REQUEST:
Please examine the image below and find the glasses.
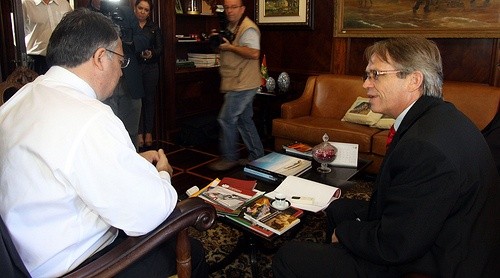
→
[106,48,131,69]
[224,4,243,9]
[361,68,401,81]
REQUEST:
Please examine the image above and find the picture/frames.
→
[333,0,500,38]
[254,0,315,32]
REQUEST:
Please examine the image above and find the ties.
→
[385,123,396,148]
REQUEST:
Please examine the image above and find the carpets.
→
[187,181,374,278]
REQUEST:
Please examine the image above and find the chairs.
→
[0,196,216,278]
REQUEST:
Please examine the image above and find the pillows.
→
[342,96,384,126]
[369,114,396,129]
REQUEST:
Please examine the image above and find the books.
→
[192,138,359,237]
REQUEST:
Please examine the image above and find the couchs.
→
[272,74,500,175]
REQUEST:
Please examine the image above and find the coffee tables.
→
[177,149,373,278]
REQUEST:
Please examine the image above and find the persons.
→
[124,1,162,149]
[82,1,145,155]
[0,8,207,278]
[272,31,500,278]
[208,0,265,171]
[19,0,75,80]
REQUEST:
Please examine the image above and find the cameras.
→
[209,30,234,48]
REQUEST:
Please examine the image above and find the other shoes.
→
[239,158,252,166]
[209,159,237,171]
[136,132,154,148]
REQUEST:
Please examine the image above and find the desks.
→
[255,88,303,144]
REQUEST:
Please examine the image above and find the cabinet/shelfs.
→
[158,0,227,142]
